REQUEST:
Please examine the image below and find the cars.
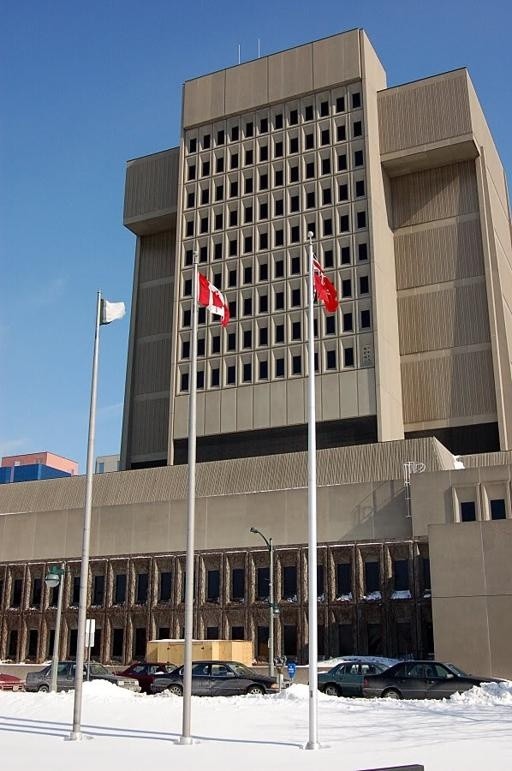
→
[318,661,391,697]
[0,660,290,696]
[362,661,509,699]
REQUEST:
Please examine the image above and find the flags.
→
[198,271,230,327]
[99,299,128,326]
[313,256,340,315]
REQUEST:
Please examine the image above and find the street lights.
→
[250,527,274,676]
[44,559,67,694]
